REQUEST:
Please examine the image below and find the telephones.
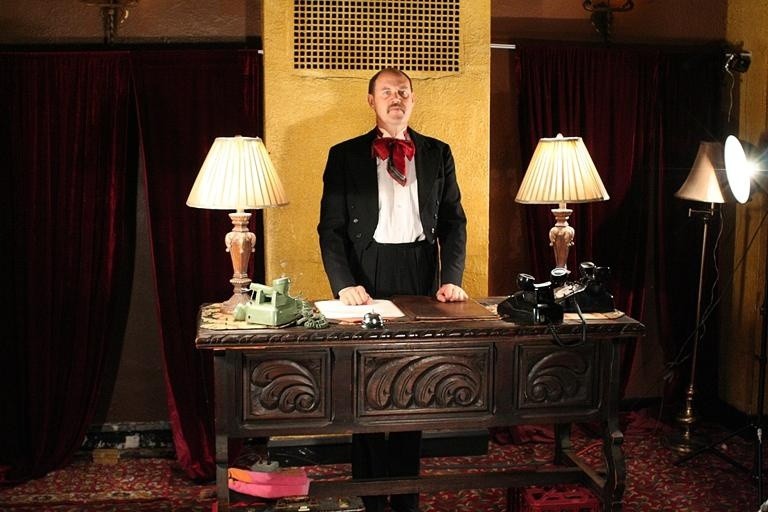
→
[496,262,616,326]
[233,276,302,326]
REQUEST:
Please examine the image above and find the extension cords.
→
[250,458,280,473]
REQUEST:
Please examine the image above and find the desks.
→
[193,291,647,511]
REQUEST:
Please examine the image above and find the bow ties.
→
[373,137,415,186]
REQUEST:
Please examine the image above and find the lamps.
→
[671,135,738,458]
[512,130,614,299]
[186,135,289,314]
[717,136,767,512]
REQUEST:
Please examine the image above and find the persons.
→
[316,67,469,510]
